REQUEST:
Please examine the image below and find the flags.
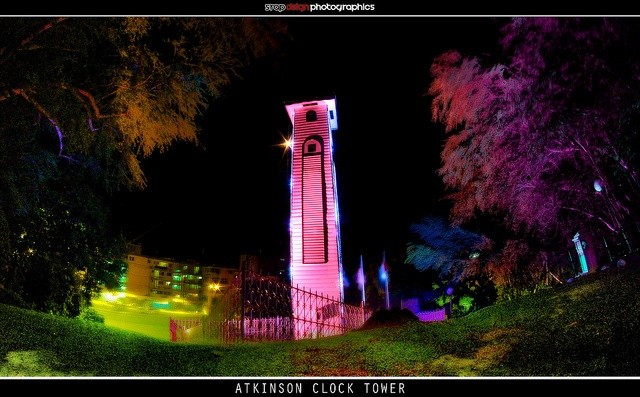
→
[378,257,391,284]
[356,267,367,291]
